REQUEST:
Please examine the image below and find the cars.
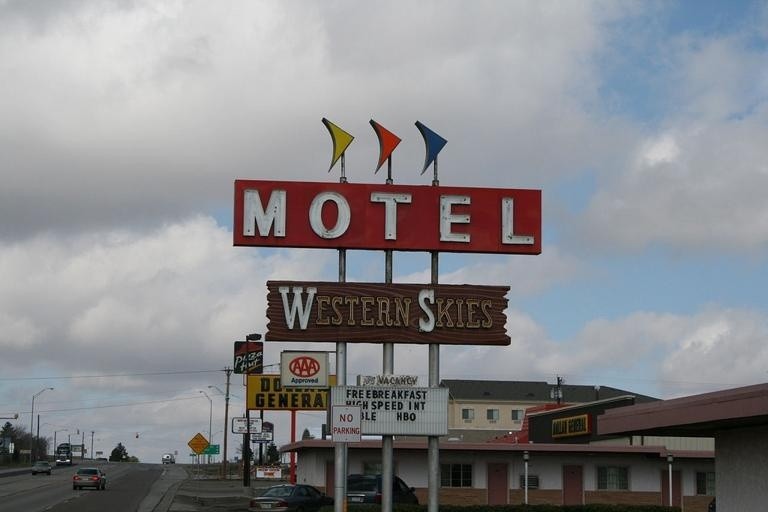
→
[248,484,333,512]
[73,467,107,490]
[31,460,51,475]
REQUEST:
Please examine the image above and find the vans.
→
[161,453,175,465]
[347,473,418,505]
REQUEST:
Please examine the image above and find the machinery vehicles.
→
[53,443,73,466]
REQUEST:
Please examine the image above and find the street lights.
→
[0,412,18,420]
[593,384,600,400]
[666,454,673,507]
[54,428,68,459]
[199,390,213,463]
[28,387,55,465]
[202,429,224,463]
[207,385,226,460]
[521,450,530,504]
